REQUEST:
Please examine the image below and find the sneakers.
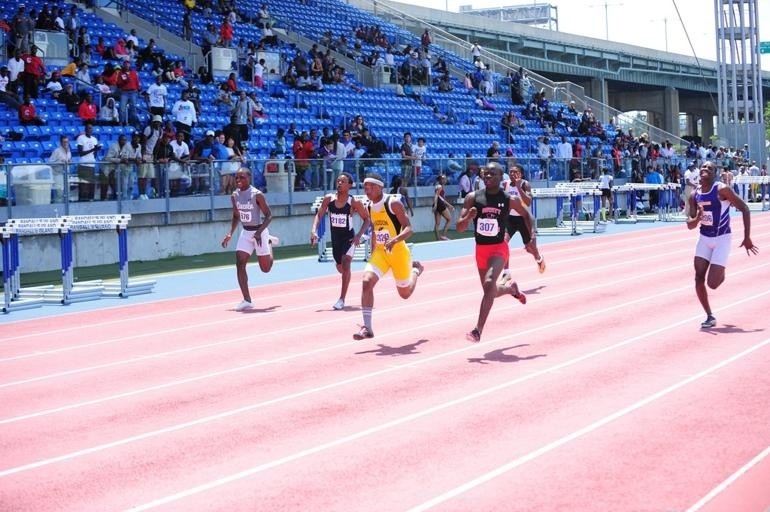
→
[333,298,345,310]
[497,272,512,285]
[701,315,717,328]
[234,300,255,311]
[269,235,280,246]
[137,194,149,200]
[509,280,527,305]
[465,328,483,342]
[353,326,374,340]
[440,235,451,242]
[413,260,425,277]
[536,255,546,273]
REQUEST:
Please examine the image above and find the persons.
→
[431,174,456,240]
[686,161,759,329]
[455,162,537,343]
[2,1,770,220]
[310,173,370,309]
[222,167,278,312]
[353,171,424,340]
[501,165,546,274]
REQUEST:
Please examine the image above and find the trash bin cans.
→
[263,161,298,192]
[12,166,55,206]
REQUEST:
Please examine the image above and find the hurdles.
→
[0,214,156,313]
[529,182,610,235]
[731,175,770,211]
[311,194,405,262]
[611,182,688,224]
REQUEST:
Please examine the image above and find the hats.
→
[206,130,215,137]
[151,114,163,124]
[132,131,142,136]
